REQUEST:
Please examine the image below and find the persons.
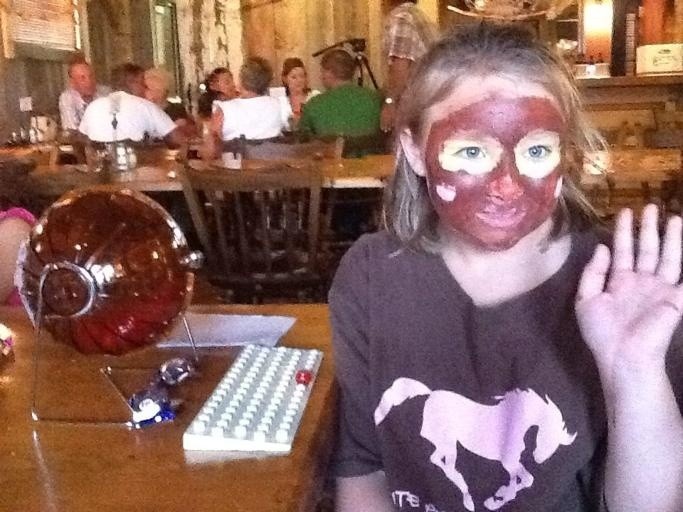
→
[59,60,112,131]
[198,67,235,120]
[379,0,438,134]
[211,56,285,141]
[143,65,189,126]
[281,57,323,117]
[0,158,38,307]
[77,63,182,150]
[327,19,683,512]
[296,50,383,159]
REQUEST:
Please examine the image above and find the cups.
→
[222,150,243,171]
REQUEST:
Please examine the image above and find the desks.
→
[1,302,340,512]
[28,148,683,196]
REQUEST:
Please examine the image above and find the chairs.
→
[239,133,344,248]
[174,152,323,303]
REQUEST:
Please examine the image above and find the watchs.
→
[383,96,395,105]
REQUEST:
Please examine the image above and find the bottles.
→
[575,53,605,64]
